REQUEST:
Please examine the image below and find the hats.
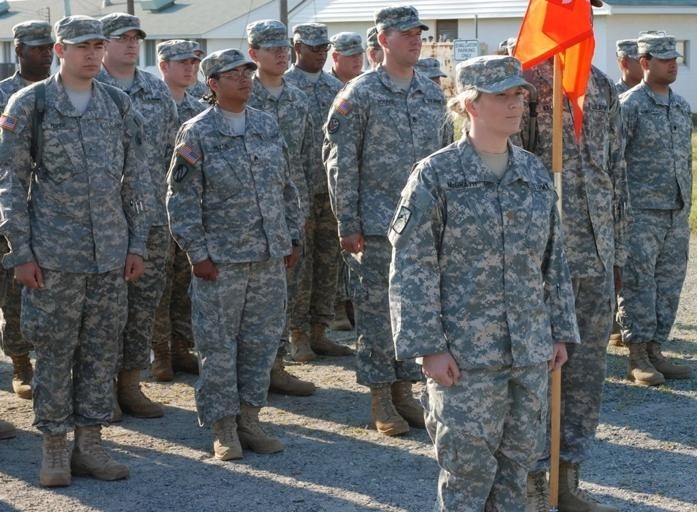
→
[637,31,683,59]
[54,14,111,46]
[616,39,639,60]
[412,58,448,78]
[367,25,382,48]
[157,39,201,61]
[12,21,56,48]
[375,5,429,31]
[188,40,204,56]
[201,48,257,79]
[292,23,334,46]
[247,19,294,48]
[330,32,365,56]
[99,12,147,41]
[455,55,536,94]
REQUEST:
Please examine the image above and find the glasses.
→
[108,34,144,46]
[298,42,331,53]
[216,68,255,79]
[253,45,291,53]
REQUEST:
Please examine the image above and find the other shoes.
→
[0,419,16,439]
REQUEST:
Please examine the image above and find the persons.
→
[388,1,696,511]
[0,5,454,487]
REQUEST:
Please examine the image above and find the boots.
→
[38,434,72,486]
[527,469,549,508]
[646,341,694,379]
[608,318,623,345]
[11,355,35,398]
[212,415,244,460]
[547,460,601,508]
[288,332,314,363]
[150,341,174,382]
[235,403,285,455]
[627,342,666,385]
[310,322,351,357]
[71,424,129,481]
[369,384,410,435]
[117,369,165,418]
[269,348,316,397]
[171,337,200,375]
[389,381,426,429]
[105,381,123,422]
[331,304,353,332]
